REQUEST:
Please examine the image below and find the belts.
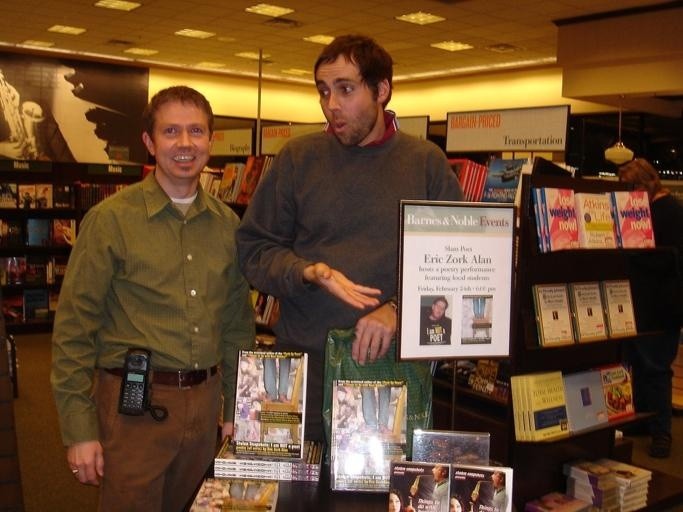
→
[111,367,219,388]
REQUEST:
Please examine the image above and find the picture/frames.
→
[394,198,518,358]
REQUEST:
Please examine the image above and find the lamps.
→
[603,96,635,167]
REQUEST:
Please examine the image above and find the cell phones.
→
[119,349,153,417]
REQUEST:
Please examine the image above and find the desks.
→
[180,436,683,512]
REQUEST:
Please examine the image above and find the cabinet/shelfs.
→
[515,168,659,444]
[186,151,561,344]
[1,157,145,334]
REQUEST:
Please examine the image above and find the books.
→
[532,280,638,347]
[191,351,513,512]
[0,182,128,323]
[510,363,635,442]
[448,157,655,252]
[523,459,653,511]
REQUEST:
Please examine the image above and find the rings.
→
[72,469,78,474]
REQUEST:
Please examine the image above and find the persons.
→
[421,297,451,345]
[50,87,254,512]
[618,158,683,458]
[237,35,465,461]
